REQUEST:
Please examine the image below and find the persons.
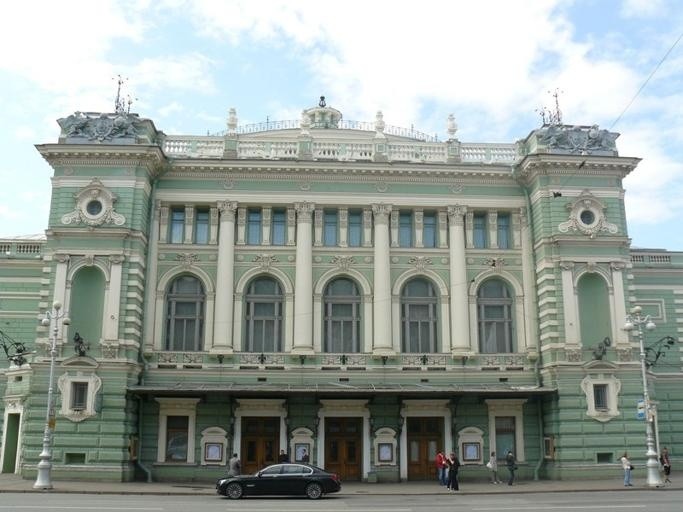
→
[506,451,516,486]
[296,448,306,461]
[489,451,503,485]
[435,448,461,492]
[620,451,634,487]
[659,446,672,484]
[228,453,241,477]
[279,449,289,464]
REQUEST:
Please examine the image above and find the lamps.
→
[491,260,496,268]
[553,192,561,198]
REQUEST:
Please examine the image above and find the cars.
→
[166,432,187,461]
[215,462,341,500]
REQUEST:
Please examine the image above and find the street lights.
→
[622,304,666,488]
[33,300,71,490]
